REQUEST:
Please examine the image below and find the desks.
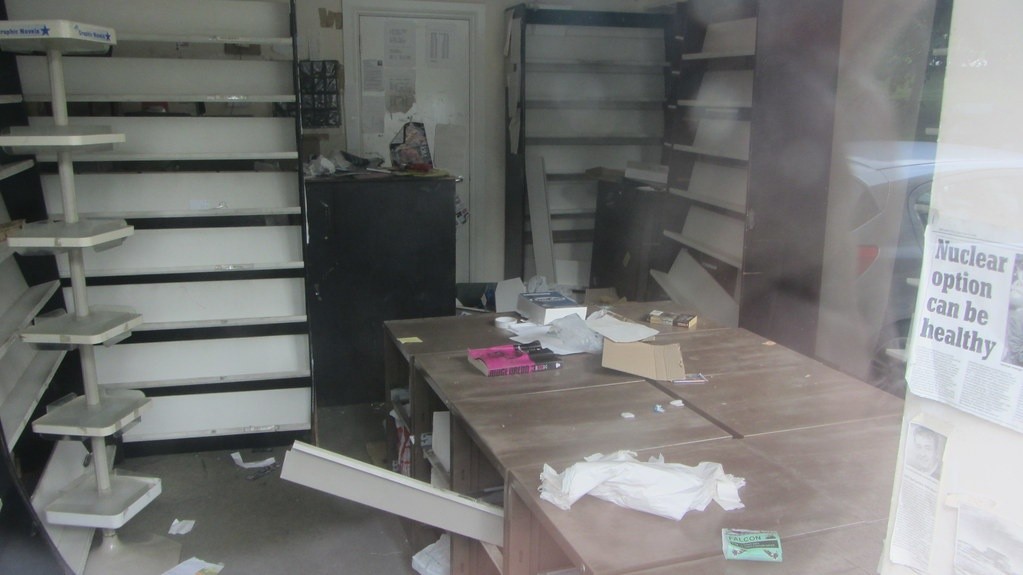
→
[383,300,1023,575]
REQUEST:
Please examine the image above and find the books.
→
[466,340,562,378]
[513,290,588,325]
[645,308,697,327]
[624,159,670,184]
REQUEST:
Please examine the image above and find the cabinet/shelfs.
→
[18,38,311,443]
[503,4,684,295]
[305,170,456,406]
[651,15,759,326]
[0,94,120,575]
[589,178,689,302]
[0,17,184,575]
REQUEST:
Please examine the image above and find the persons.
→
[905,427,943,480]
